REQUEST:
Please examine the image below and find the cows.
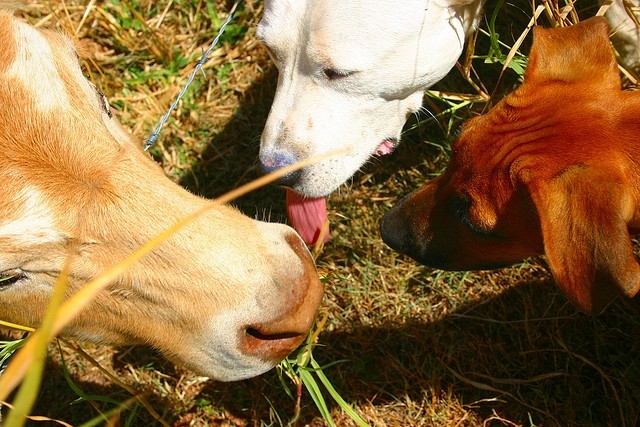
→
[0,10,324,384]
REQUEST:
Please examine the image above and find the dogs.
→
[256,0,482,246]
[380,16,640,317]
[606,0,640,74]
